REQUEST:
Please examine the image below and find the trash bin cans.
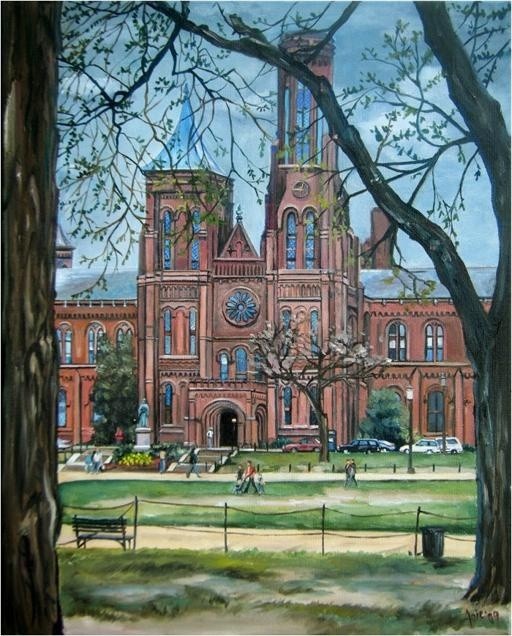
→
[420,526,444,558]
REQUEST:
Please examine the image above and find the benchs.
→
[72,514,135,550]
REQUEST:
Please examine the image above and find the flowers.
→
[119,453,154,468]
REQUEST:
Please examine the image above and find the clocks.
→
[291,179,310,199]
